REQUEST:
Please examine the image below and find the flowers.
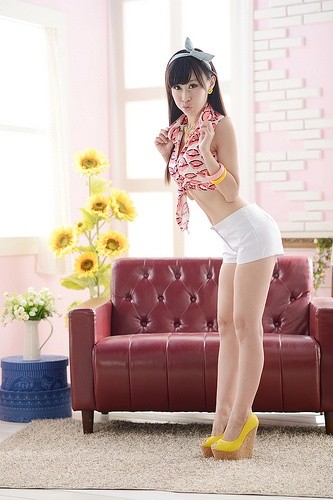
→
[40,148,138,333]
[0,286,65,328]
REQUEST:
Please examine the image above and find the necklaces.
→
[185,123,195,133]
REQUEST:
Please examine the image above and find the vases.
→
[23,318,53,362]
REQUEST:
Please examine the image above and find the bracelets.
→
[206,163,227,184]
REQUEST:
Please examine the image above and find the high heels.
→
[200,430,225,458]
[211,414,259,460]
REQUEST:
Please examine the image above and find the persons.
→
[155,37,284,459]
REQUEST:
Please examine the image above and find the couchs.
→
[69,258,333,435]
